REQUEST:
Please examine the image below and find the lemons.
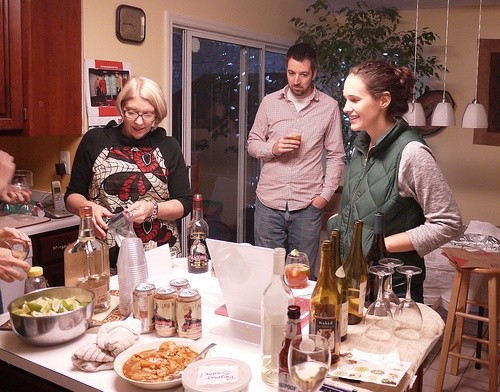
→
[12,297,87,317]
[290,248,300,257]
[300,267,309,273]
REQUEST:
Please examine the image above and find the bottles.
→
[25,266,46,295]
[261,246,296,385]
[278,305,308,392]
[343,219,368,326]
[63,206,110,314]
[309,228,347,365]
[187,193,209,274]
[365,211,391,308]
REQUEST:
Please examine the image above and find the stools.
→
[435,258,500,392]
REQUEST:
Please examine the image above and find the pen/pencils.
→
[324,381,358,392]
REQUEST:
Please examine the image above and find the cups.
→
[285,252,309,289]
[0,199,36,221]
[181,356,253,392]
[0,237,30,261]
[8,169,34,192]
[116,238,150,316]
[285,129,301,150]
[287,335,331,392]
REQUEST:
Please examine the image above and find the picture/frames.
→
[472,38,500,148]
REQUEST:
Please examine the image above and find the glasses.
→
[123,108,157,123]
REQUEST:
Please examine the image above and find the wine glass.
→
[363,258,423,342]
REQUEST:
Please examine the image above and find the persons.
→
[326,60,463,304]
[0,149,32,283]
[94,73,127,106]
[247,42,345,281]
[64,77,195,277]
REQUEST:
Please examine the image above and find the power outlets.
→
[60,149,71,173]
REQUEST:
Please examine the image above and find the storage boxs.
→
[208,291,313,347]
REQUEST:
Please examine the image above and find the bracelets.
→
[146,198,158,222]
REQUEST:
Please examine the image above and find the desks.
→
[423,220,500,369]
[0,232,446,392]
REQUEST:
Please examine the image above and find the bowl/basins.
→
[6,286,95,346]
[113,338,210,390]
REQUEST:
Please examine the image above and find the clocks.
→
[115,3,147,43]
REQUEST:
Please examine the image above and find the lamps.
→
[430,0,457,126]
[401,0,426,127]
[462,0,488,129]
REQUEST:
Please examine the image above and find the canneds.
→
[133,278,203,340]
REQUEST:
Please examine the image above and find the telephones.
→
[44,181,75,219]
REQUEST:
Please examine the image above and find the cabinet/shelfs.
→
[30,226,79,287]
[0,0,83,134]
